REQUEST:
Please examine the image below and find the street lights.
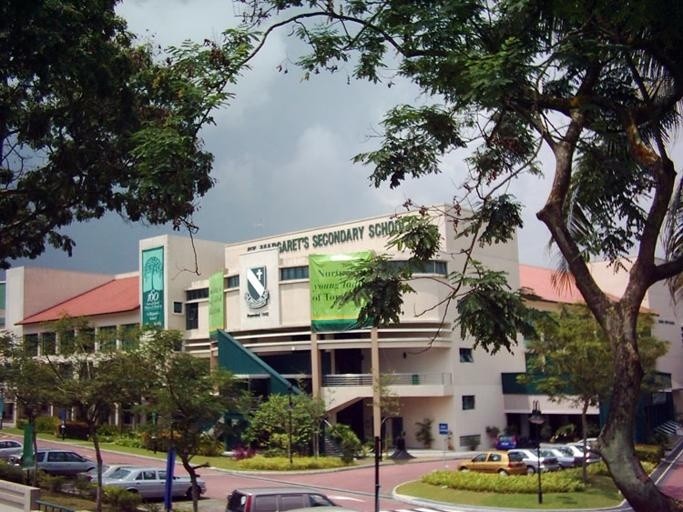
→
[373,413,416,511]
[529,399,544,503]
[284,382,293,464]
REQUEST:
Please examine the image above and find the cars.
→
[458,431,602,476]
[0,438,206,501]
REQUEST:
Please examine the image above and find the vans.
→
[225,487,336,511]
